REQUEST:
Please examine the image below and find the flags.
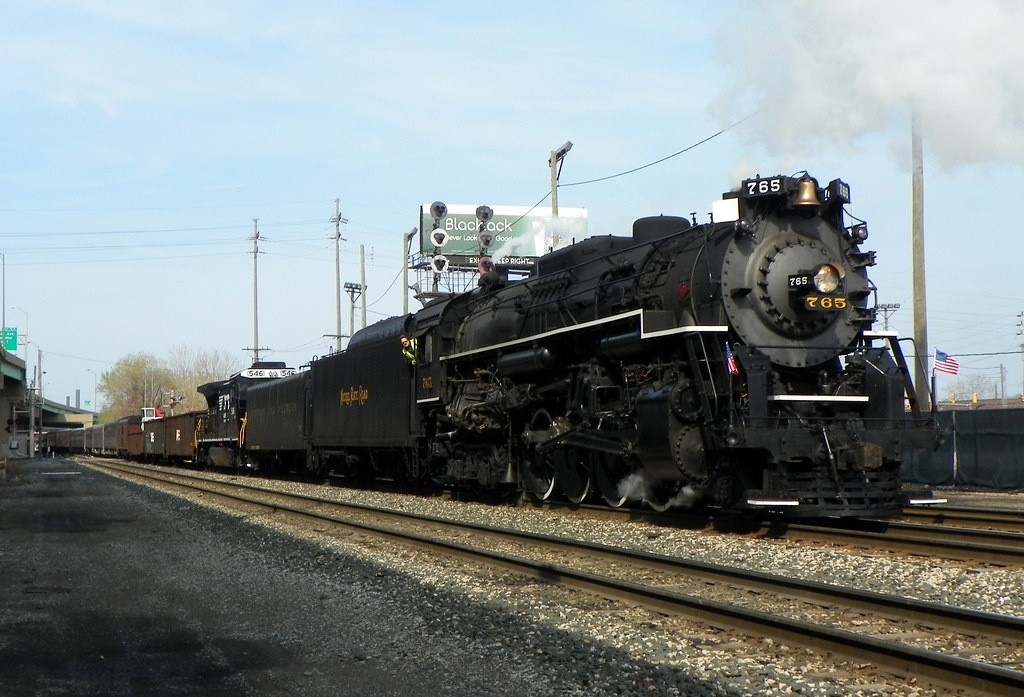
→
[934,348,959,375]
[726,342,739,375]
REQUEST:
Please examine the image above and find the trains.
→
[43,170,956,539]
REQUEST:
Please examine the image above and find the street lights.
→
[87,366,96,413]
[11,306,29,360]
